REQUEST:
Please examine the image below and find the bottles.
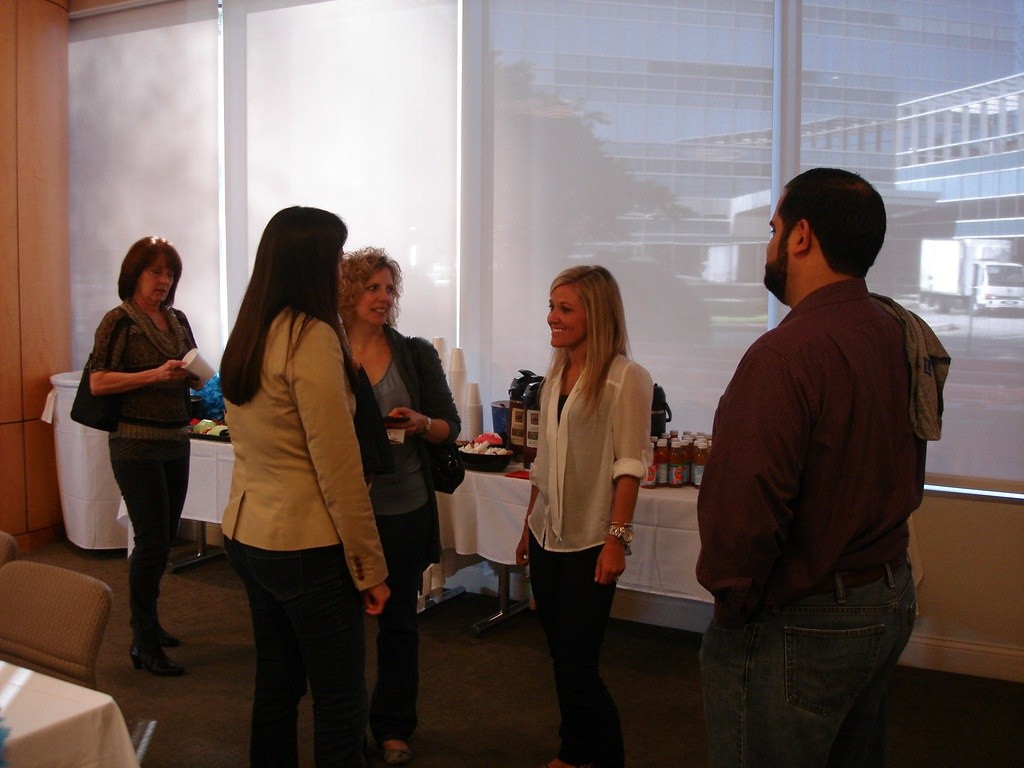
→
[632,429,715,490]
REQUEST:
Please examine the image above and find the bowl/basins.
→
[456,444,516,473]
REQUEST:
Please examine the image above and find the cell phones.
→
[381,415,411,423]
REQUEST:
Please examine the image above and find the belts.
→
[809,552,907,594]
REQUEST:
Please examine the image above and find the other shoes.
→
[380,739,413,765]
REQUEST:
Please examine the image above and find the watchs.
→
[609,526,634,543]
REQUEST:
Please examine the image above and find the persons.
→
[220,208,390,768]
[696,168,927,768]
[89,237,206,674]
[517,266,654,768]
[338,251,462,764]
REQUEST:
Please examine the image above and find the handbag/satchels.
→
[71,307,129,433]
[429,442,465,495]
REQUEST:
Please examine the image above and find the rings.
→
[615,576,620,582]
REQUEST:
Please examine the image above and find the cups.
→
[181,348,215,381]
[431,336,484,444]
[490,400,511,444]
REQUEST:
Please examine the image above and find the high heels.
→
[130,617,179,647]
[130,637,183,677]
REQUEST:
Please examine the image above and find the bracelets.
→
[611,521,633,528]
[415,417,432,436]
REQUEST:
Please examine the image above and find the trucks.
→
[917,237,1024,318]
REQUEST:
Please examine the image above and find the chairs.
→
[0,531,112,687]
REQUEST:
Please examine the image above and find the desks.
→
[0,662,141,767]
[115,435,1023,686]
